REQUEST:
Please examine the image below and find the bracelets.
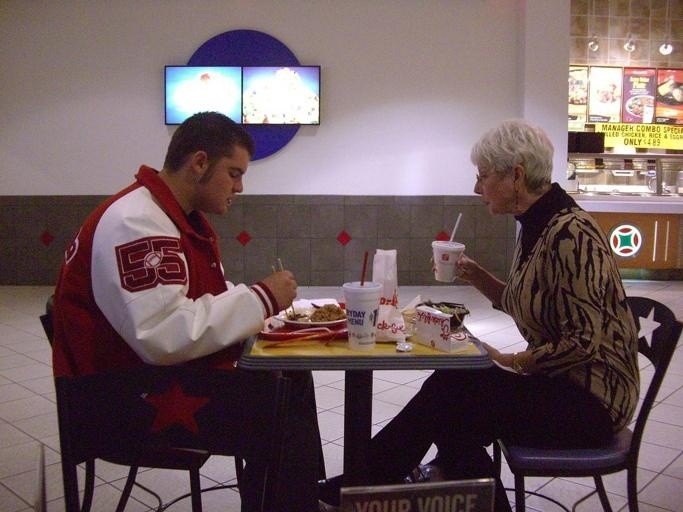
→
[512,352,525,374]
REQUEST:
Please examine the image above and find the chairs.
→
[40,295,245,511]
[493,296,683,511]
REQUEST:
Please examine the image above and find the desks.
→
[248,309,495,471]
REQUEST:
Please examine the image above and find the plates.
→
[624,95,654,119]
[272,304,352,326]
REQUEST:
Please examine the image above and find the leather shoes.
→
[318,474,343,506]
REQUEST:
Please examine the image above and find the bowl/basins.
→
[415,303,470,328]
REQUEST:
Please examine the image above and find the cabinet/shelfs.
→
[568,151,682,272]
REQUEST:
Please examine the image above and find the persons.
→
[53,112,326,512]
[313,118,640,512]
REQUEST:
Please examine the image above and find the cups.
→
[342,280,383,351]
[431,240,465,282]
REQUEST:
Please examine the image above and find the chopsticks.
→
[270,257,296,321]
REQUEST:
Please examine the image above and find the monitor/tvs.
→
[241,64,322,126]
[164,64,242,127]
[568,131,604,166]
